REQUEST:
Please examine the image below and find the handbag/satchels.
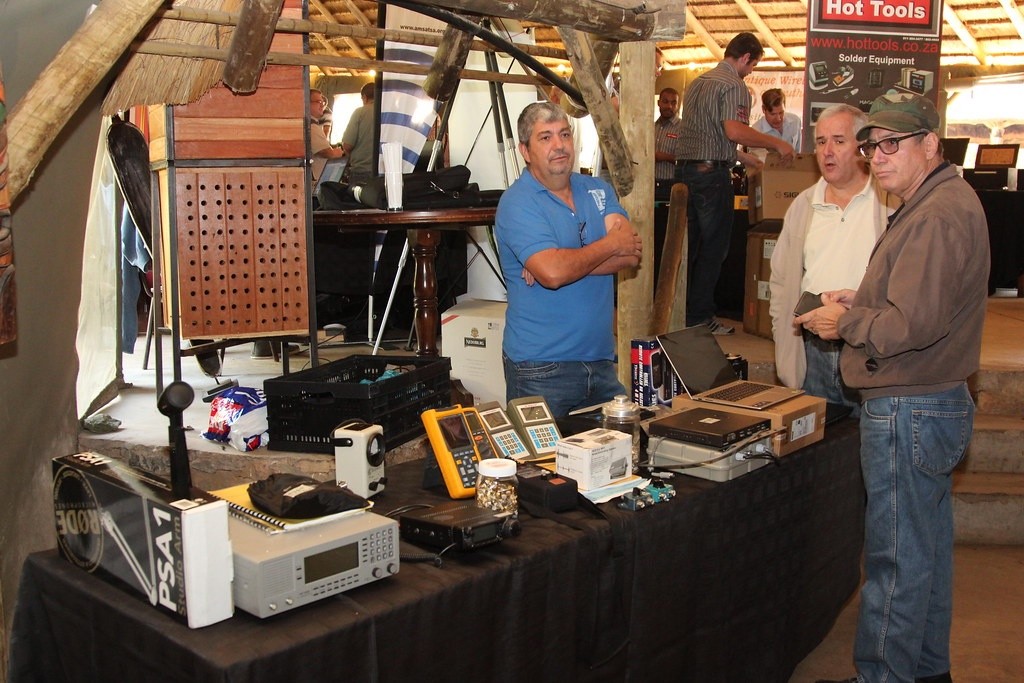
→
[201,386,269,453]
[320,164,483,210]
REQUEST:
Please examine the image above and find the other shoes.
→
[814,678,858,683]
[914,671,953,683]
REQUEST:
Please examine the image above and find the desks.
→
[313,207,498,374]
[973,190,1024,297]
[11,403,866,683]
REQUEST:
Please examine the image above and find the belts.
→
[675,160,731,170]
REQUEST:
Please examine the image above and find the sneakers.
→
[686,315,735,335]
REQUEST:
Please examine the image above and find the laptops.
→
[656,323,806,410]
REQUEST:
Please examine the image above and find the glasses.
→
[857,131,929,160]
[310,99,326,108]
[577,221,588,249]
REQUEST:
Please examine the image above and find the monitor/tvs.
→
[940,136,970,166]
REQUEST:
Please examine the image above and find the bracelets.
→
[675,161,677,165]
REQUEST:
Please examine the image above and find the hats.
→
[856,93,940,142]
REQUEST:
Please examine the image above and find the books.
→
[206,482,374,535]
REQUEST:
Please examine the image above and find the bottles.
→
[475,457,520,539]
[601,394,641,473]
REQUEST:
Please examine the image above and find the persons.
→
[769,105,901,420]
[740,89,802,176]
[342,82,375,185]
[794,92,990,683]
[654,88,682,298]
[674,32,798,335]
[309,88,342,180]
[494,103,643,410]
[612,47,663,112]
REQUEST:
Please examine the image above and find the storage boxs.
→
[672,391,827,458]
[264,355,452,456]
[744,153,903,340]
[557,429,632,491]
[441,294,507,410]
[52,451,236,630]
